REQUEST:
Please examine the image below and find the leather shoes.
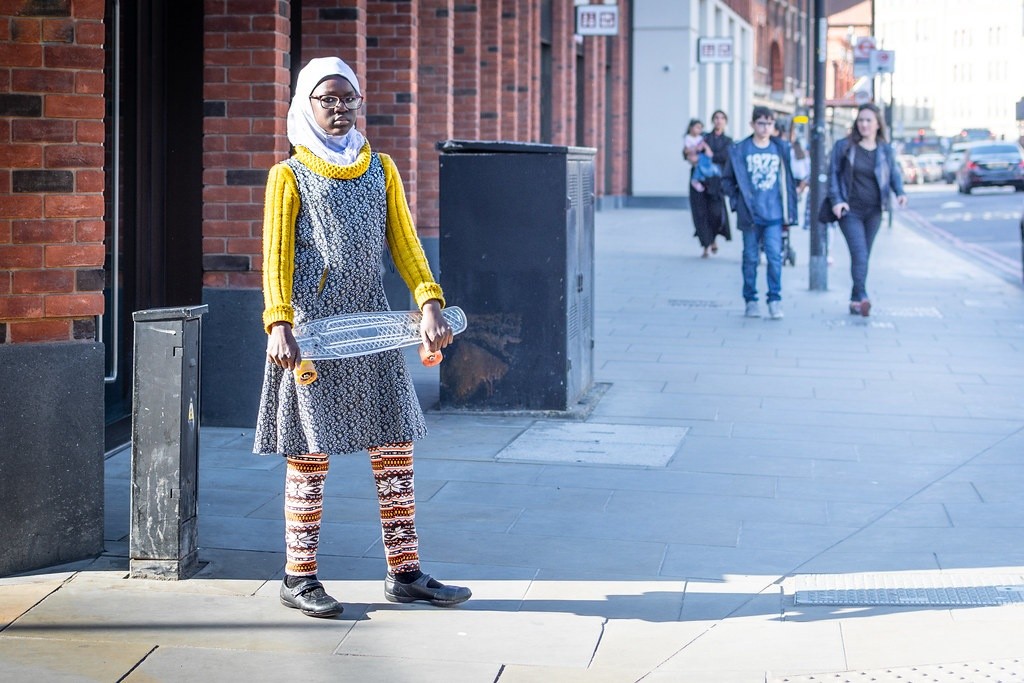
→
[383,568,472,608]
[280,573,343,617]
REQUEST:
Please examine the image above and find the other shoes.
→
[849,302,862,315]
[745,301,761,318]
[698,251,709,258]
[860,298,871,316]
[768,301,783,319]
[710,241,719,253]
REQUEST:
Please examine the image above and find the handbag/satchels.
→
[819,197,840,223]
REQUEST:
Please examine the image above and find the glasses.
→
[756,121,774,127]
[306,95,363,112]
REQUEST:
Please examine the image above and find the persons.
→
[681,117,714,192]
[760,118,812,267]
[825,103,908,317]
[251,56,471,618]
[682,111,735,259]
[718,106,800,321]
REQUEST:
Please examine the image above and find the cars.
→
[895,153,947,183]
[958,141,1024,194]
[945,143,970,183]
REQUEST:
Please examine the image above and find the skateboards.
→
[781,226,796,267]
[291,305,468,385]
